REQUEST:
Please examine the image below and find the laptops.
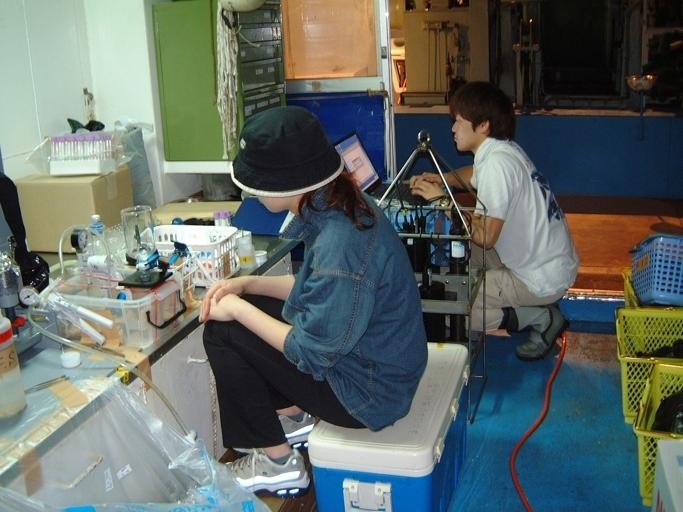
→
[331,131,428,202]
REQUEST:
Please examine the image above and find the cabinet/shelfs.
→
[151,0,285,159]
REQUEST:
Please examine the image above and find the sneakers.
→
[232,411,314,454]
[516,304,565,361]
[221,451,310,497]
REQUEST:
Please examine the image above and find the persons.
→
[409,82,578,360]
[199,106,427,499]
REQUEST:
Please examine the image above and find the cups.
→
[118,204,158,265]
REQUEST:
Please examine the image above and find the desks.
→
[0,234,303,512]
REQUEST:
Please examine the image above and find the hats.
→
[231,106,343,197]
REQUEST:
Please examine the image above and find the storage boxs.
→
[649,439,682,512]
[305,343,471,512]
[48,266,186,349]
[15,162,134,252]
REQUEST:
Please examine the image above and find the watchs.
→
[439,198,450,213]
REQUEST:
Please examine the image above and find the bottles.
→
[88,214,109,257]
[213,210,232,228]
[50,134,113,161]
[238,237,255,268]
[0,309,26,419]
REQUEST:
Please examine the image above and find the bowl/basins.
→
[626,75,656,91]
